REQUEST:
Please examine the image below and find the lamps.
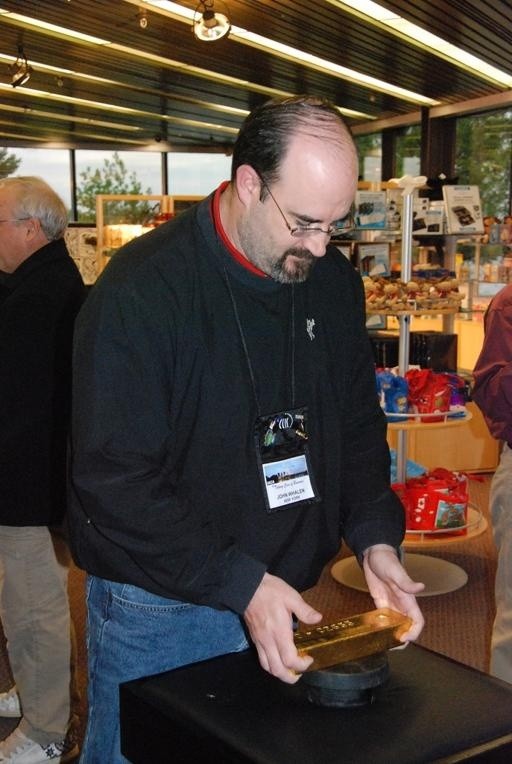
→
[190,0,232,45]
[7,42,34,87]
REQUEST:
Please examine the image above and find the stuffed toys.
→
[355,273,466,320]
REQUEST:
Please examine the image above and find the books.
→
[337,176,486,278]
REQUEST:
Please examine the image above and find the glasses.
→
[254,166,357,241]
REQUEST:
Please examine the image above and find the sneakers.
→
[1,727,80,764]
[0,683,23,718]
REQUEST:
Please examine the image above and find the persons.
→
[1,173,91,764]
[59,92,426,764]
[468,276,510,684]
